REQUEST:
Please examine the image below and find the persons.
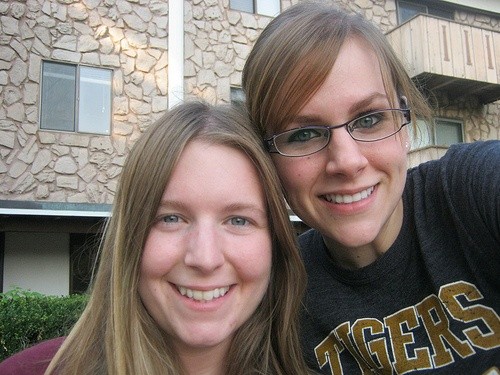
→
[241,0,500,375]
[0,101,320,375]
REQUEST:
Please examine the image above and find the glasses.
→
[263,106,411,158]
